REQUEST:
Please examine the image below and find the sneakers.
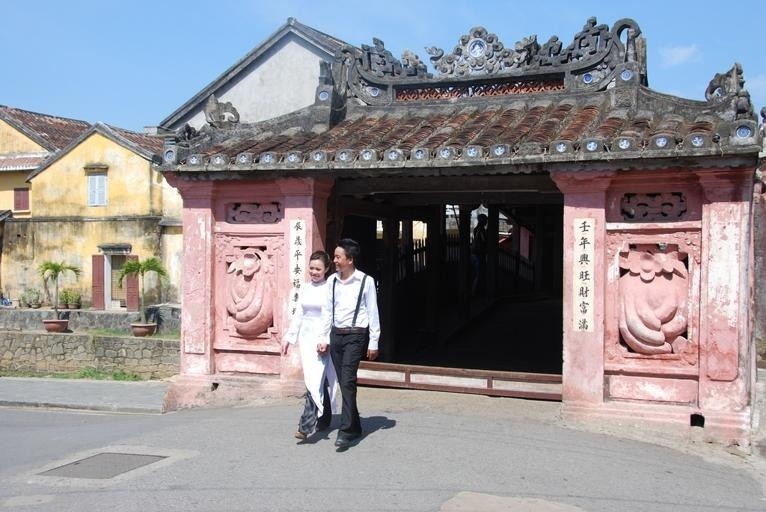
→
[295,431,307,440]
[314,420,328,431]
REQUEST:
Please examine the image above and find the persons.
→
[470,214,495,298]
[317,239,381,447]
[279,251,334,441]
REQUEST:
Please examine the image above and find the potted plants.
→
[116,255,168,336]
[12,258,83,332]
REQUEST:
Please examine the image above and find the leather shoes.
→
[335,437,352,447]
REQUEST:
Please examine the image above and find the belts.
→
[333,327,368,335]
